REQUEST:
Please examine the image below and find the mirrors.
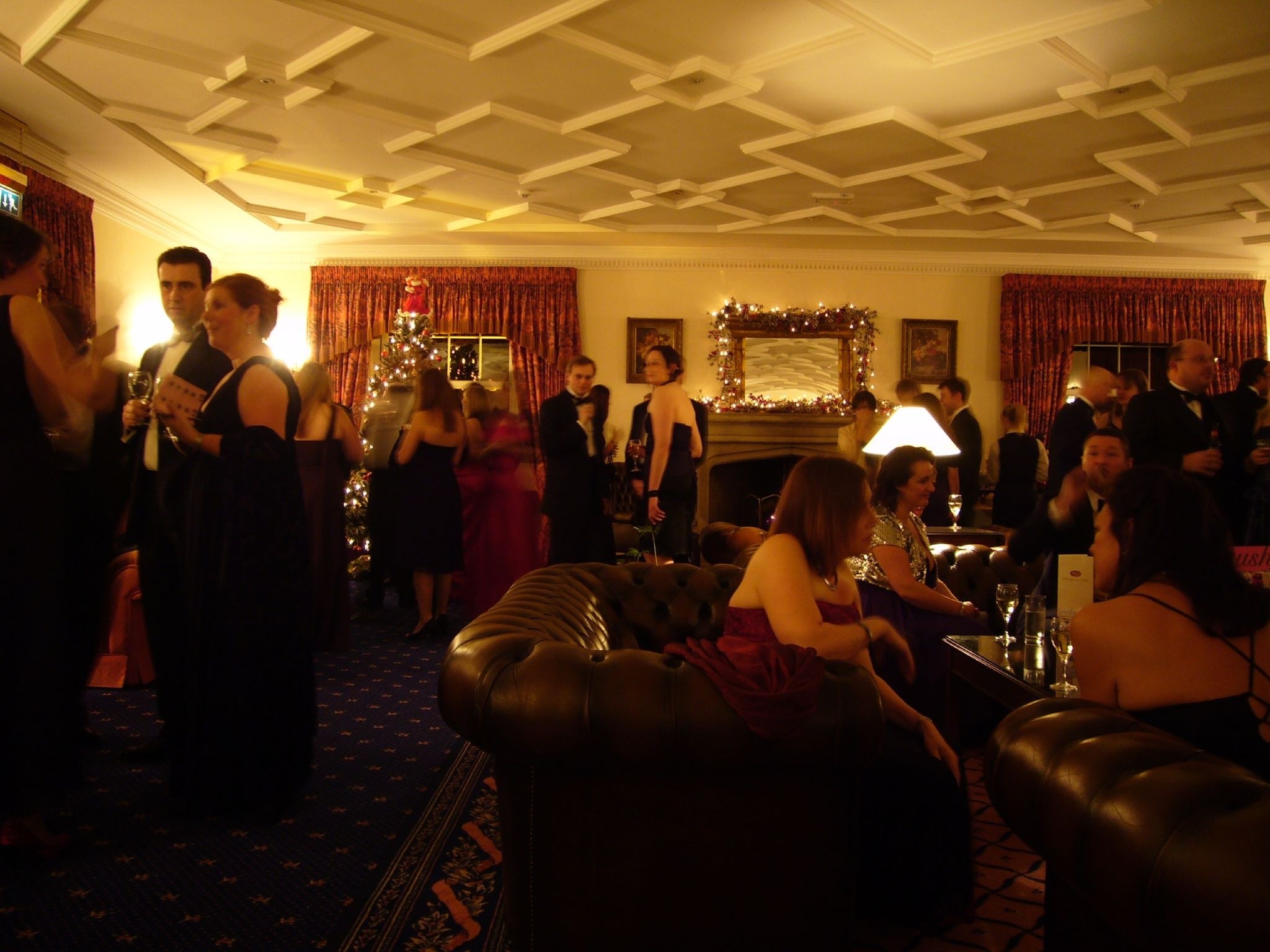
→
[742,337,839,403]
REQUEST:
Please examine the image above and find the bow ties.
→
[573,396,591,405]
[1178,390,1208,403]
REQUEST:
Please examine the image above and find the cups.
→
[1024,595,1046,647]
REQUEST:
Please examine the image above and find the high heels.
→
[405,617,436,643]
[436,615,448,629]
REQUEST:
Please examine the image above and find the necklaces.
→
[825,570,837,589]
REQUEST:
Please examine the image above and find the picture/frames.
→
[902,318,958,384]
[627,317,683,383]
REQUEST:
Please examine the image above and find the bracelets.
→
[194,431,204,452]
[645,489,661,498]
[856,620,875,644]
[914,716,933,729]
[959,601,966,616]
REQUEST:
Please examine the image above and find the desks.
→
[924,525,1007,547]
[941,634,1053,739]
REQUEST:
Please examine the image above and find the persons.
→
[155,273,311,823]
[663,457,972,930]
[835,339,1270,636]
[624,341,708,567]
[290,362,366,655]
[356,368,543,640]
[1071,460,1270,778]
[530,354,621,568]
[121,247,234,826]
[845,446,990,726]
[0,202,129,884]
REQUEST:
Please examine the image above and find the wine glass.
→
[127,371,153,427]
[995,584,1019,643]
[1257,441,1266,465]
[1050,616,1078,692]
[948,494,962,530]
[629,439,641,472]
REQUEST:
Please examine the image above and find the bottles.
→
[1207,421,1221,476]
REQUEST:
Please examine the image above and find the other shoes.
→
[118,741,175,764]
[358,590,384,606]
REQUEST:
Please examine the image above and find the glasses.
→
[1174,355,1215,365]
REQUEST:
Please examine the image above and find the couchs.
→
[986,699,1270,952]
[438,562,886,952]
[928,544,1008,613]
